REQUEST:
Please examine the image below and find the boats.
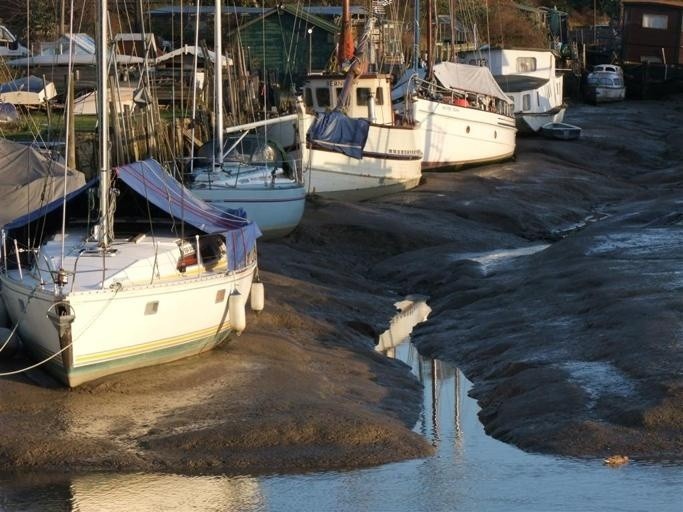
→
[586,62,626,103]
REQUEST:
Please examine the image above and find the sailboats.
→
[187,0,305,241]
[1,46,265,389]
[260,1,423,190]
[455,48,565,135]
[383,0,517,171]
